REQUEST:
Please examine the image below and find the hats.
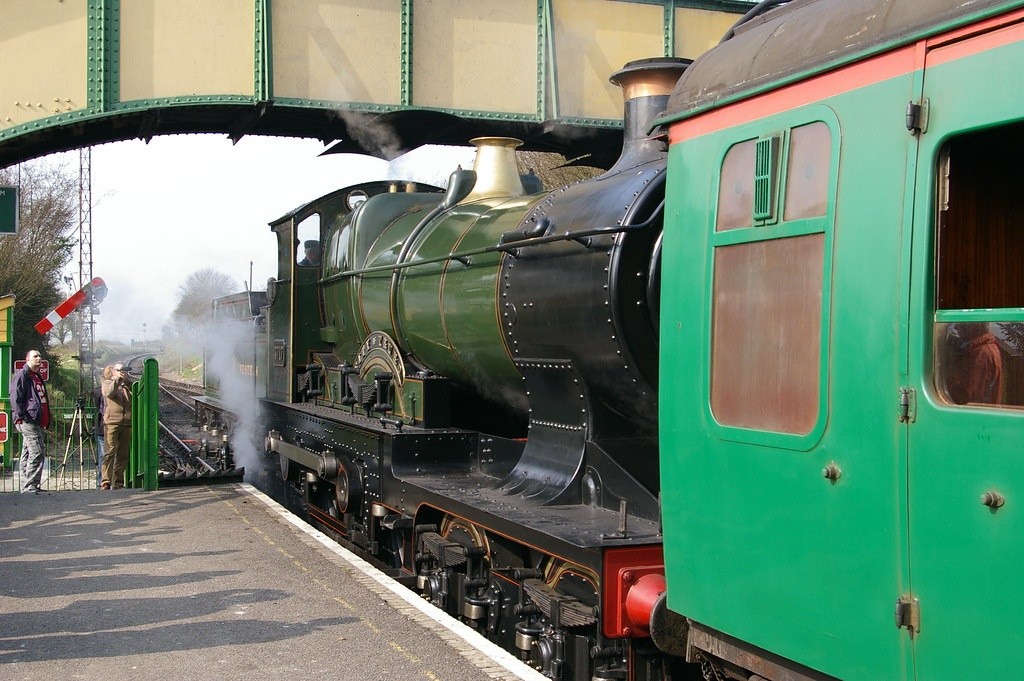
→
[304,240,318,248]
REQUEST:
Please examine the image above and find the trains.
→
[197,1,1022,680]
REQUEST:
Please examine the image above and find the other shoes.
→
[101,484,125,490]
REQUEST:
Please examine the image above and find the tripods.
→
[57,359,99,493]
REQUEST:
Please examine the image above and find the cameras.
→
[119,366,132,382]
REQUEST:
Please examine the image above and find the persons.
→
[937,321,1002,406]
[93,362,133,490]
[10,350,51,493]
